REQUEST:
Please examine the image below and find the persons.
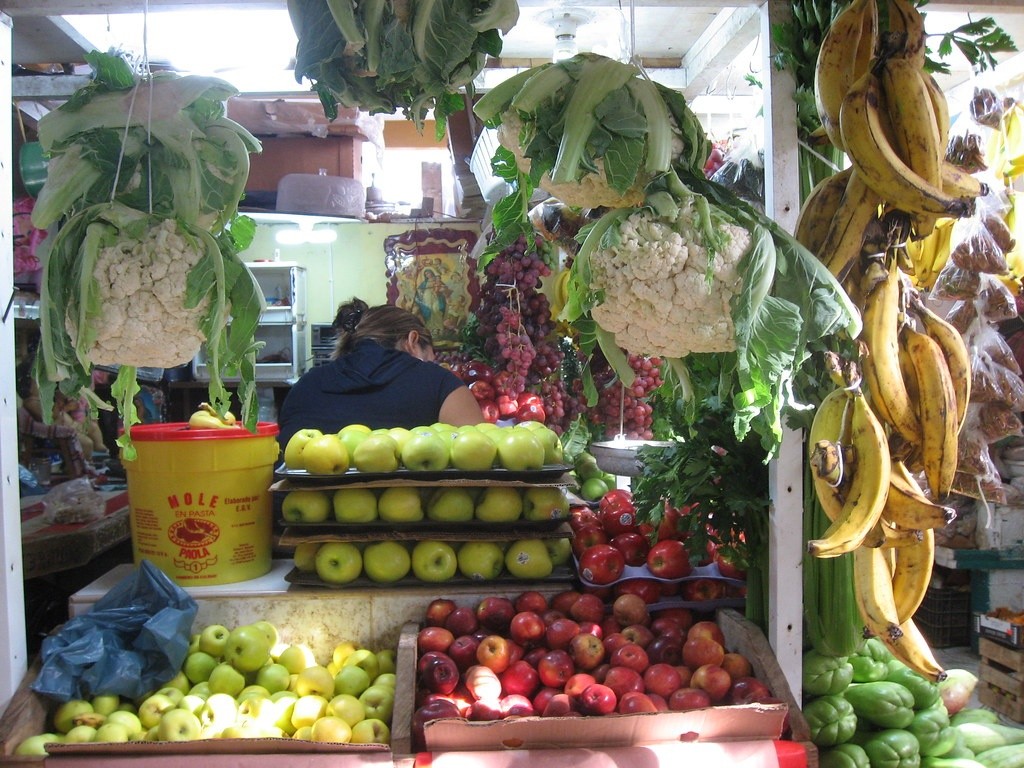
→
[274,296,484,455]
[16,375,108,465]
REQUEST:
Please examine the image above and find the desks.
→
[937,545,1024,657]
[19,484,132,580]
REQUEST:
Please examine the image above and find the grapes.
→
[434,232,663,441]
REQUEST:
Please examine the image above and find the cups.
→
[29,456,53,486]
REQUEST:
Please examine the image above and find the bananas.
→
[991,103,1024,298]
[796,0,988,683]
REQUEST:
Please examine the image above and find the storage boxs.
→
[913,585,973,649]
[241,135,363,188]
[977,613,1024,722]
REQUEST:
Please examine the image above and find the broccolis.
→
[496,94,685,212]
[587,208,754,357]
[48,129,227,370]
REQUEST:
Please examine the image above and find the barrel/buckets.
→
[116,415,279,590]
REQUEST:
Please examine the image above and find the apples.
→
[13,364,790,755]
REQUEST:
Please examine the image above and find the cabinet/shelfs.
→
[193,262,307,381]
